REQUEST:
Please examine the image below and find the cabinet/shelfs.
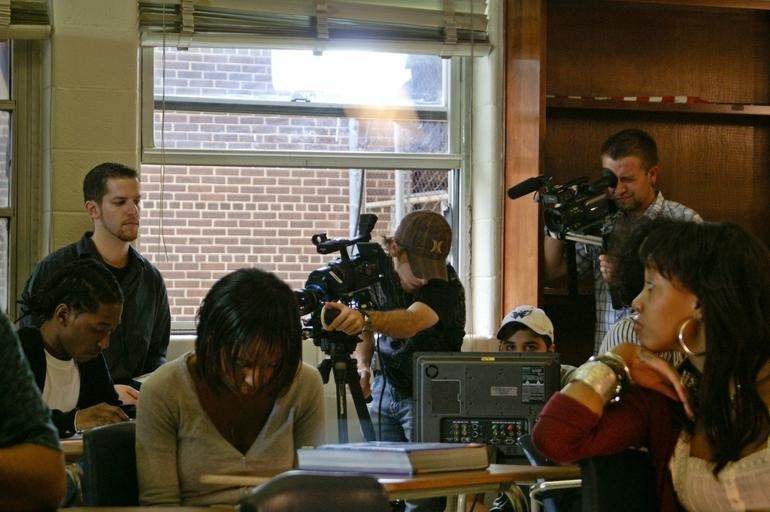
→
[502,2,770,326]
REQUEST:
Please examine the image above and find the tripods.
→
[317,356,375,444]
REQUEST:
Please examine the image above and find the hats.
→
[395,210,452,282]
[497,305,555,345]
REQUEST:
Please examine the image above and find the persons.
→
[322,210,467,509]
[17,161,171,410]
[532,220,769,511]
[542,128,706,369]
[136,270,327,512]
[0,306,68,511]
[459,306,581,511]
[2,254,136,442]
[594,212,698,389]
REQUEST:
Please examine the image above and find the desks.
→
[195,459,580,512]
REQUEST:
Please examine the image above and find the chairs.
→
[241,470,388,510]
[580,452,655,510]
[84,421,140,505]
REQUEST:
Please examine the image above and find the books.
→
[292,439,490,478]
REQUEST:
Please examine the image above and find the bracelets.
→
[566,348,635,406]
